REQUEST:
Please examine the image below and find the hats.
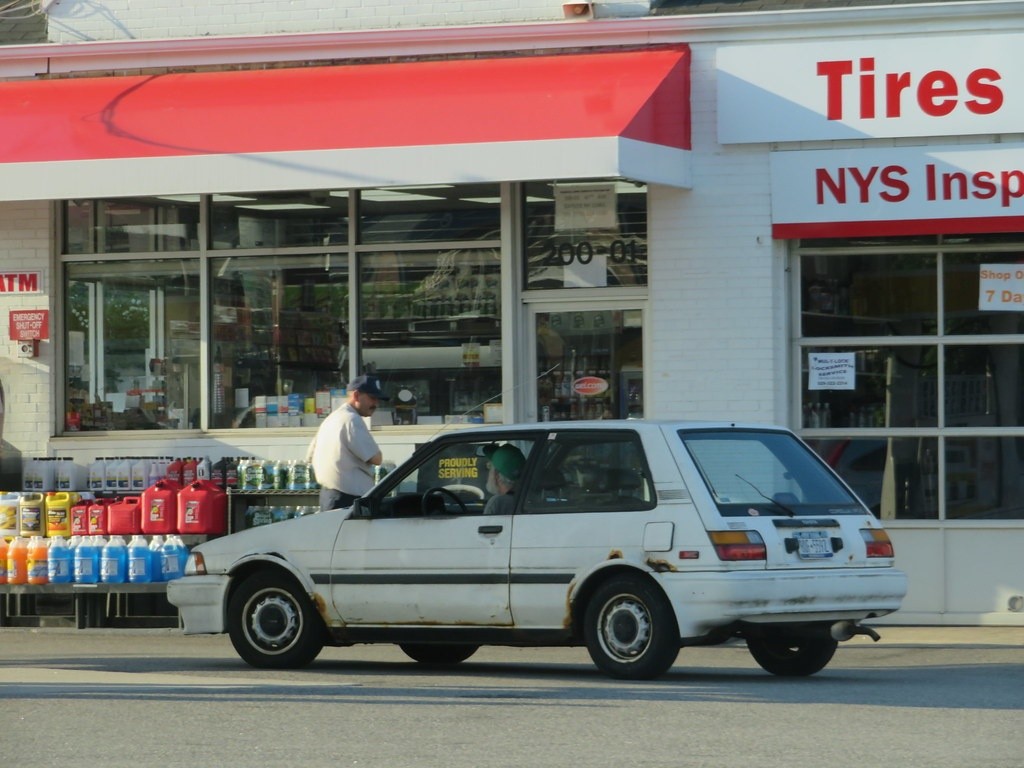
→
[347,375,391,402]
[482,443,526,480]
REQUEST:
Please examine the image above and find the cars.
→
[164,420,910,681]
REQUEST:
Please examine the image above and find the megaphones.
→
[562,2,595,20]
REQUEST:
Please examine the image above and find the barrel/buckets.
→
[21,452,402,499]
[0,533,190,585]
[0,478,229,539]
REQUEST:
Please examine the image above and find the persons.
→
[480,443,525,517]
[303,374,382,515]
[445,304,570,422]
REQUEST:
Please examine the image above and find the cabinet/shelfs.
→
[0,452,321,628]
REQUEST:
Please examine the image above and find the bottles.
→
[409,293,496,320]
[803,401,831,430]
[538,368,613,421]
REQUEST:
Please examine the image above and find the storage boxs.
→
[254,385,502,430]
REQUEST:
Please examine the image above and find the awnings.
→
[0,35,695,210]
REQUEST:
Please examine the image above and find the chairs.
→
[546,464,645,513]
[770,491,801,506]
